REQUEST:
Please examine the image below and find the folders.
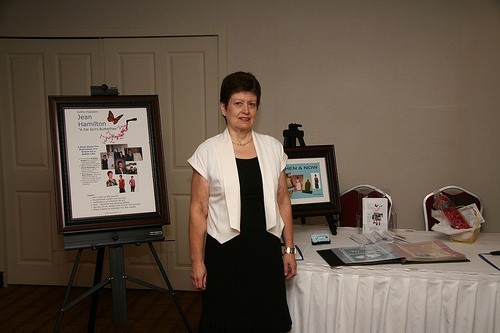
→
[315,241,471,268]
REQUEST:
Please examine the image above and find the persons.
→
[102,146,144,193]
[187,71,297,333]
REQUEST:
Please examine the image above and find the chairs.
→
[422,186,484,231]
[336,184,393,227]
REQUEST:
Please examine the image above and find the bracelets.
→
[284,247,295,254]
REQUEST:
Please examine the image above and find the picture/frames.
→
[48,94,171,235]
[283,144,343,218]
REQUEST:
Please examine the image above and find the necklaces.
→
[232,138,253,146]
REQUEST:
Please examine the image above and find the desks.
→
[281,223,500,333]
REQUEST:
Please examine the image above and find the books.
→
[281,242,303,261]
[317,240,471,268]
